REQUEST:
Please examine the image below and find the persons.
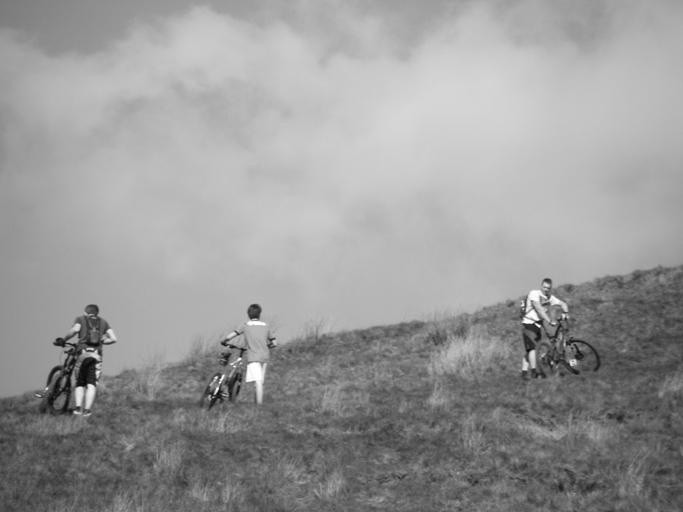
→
[519,278,570,381]
[218,303,279,406]
[55,304,117,420]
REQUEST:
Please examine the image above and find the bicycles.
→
[33,341,79,415]
[536,313,600,378]
[200,341,248,411]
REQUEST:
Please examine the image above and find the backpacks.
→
[84,314,101,347]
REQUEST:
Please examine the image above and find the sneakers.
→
[72,407,92,417]
